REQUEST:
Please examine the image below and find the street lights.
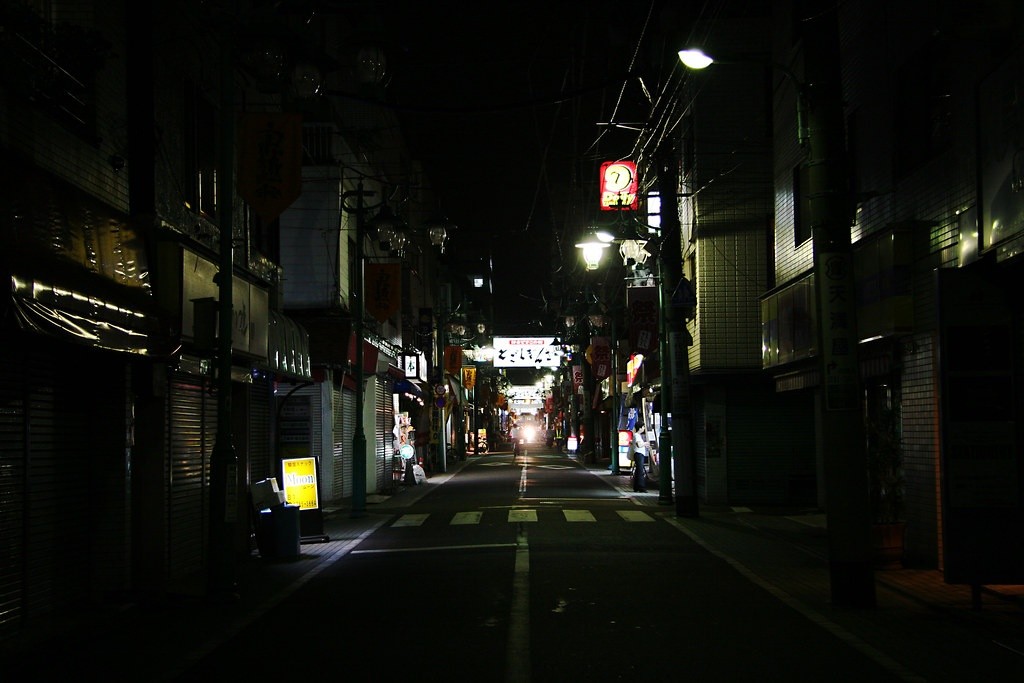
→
[473,333,496,456]
[208,1,394,612]
[352,177,457,508]
[557,278,619,475]
[573,190,673,506]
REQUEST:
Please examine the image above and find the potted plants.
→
[863,394,912,573]
